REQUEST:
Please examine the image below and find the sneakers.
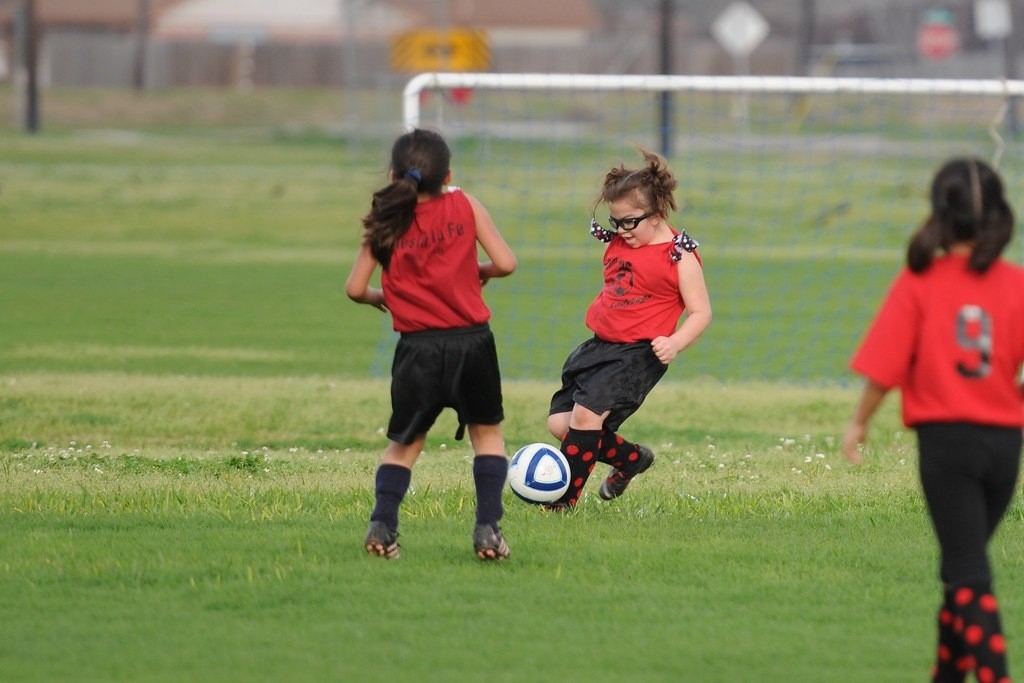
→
[472,522,510,560]
[365,521,402,561]
[599,444,654,501]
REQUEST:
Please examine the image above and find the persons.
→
[543,145,712,515]
[346,128,518,561]
[844,156,1024,683]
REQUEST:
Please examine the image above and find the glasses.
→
[608,211,658,230]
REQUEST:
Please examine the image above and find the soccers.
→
[508,443,571,506]
